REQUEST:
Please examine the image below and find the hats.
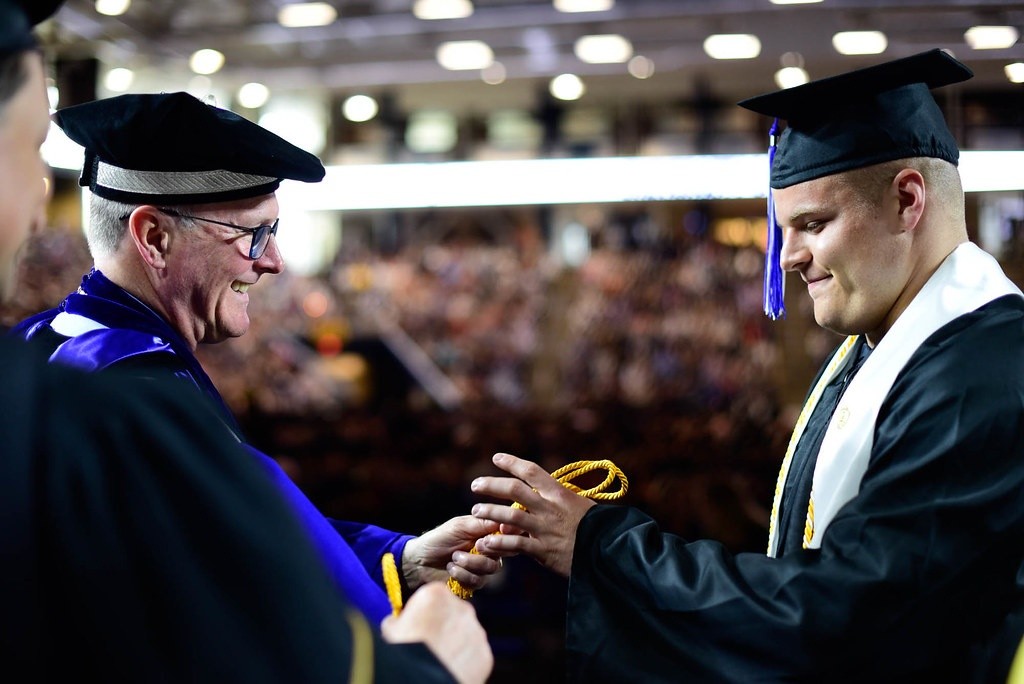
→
[734,48,974,322]
[50,91,325,205]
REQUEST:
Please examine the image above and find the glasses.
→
[118,208,280,259]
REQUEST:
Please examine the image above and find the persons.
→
[7,192,798,555]
[0,1,64,307]
[472,49,1024,682]
[0,92,505,684]
